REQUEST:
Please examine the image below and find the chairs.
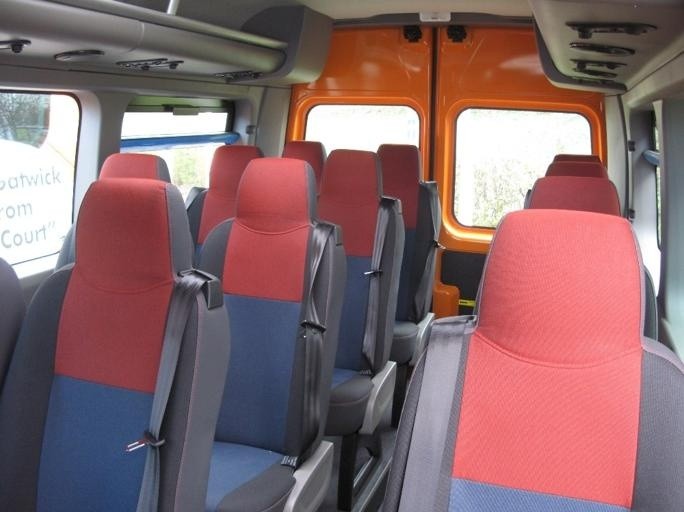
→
[52,151,173,272]
[318,148,407,511]
[280,139,328,188]
[519,151,663,344]
[378,144,446,431]
[1,176,234,512]
[193,156,350,512]
[186,144,264,269]
[381,207,681,511]
